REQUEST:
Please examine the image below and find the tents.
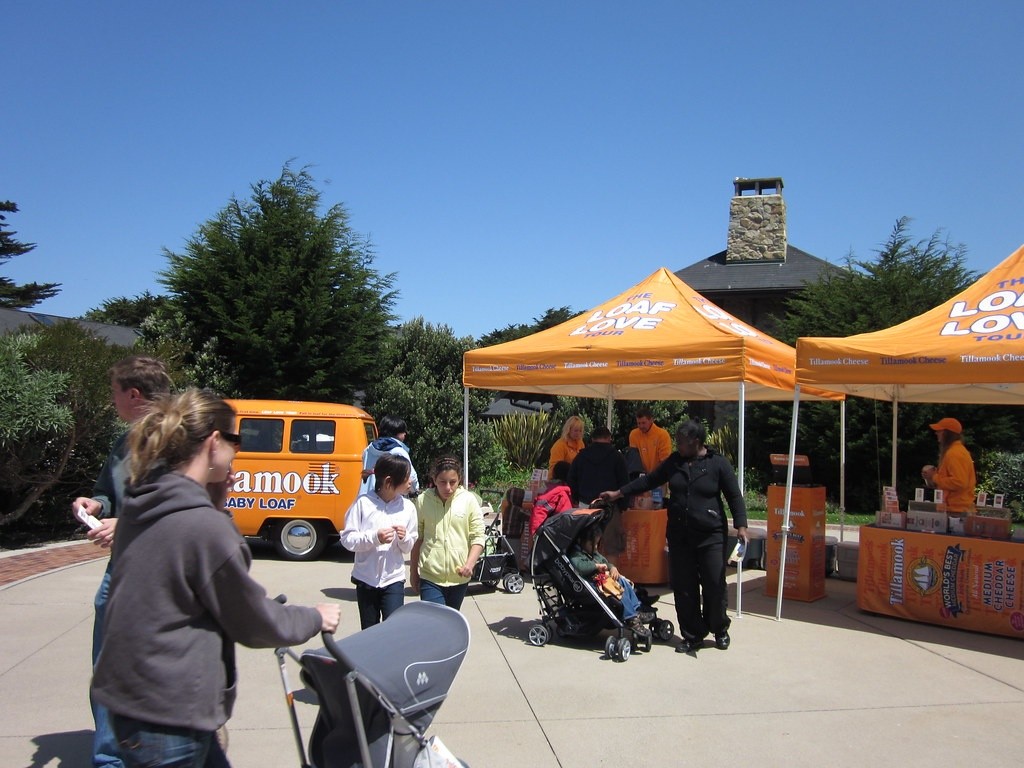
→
[775,240,1023,630]
[459,266,847,619]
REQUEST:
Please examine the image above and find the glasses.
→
[197,429,242,455]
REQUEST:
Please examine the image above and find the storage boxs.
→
[725,525,767,568]
[825,535,837,575]
[965,516,1009,538]
[907,500,948,533]
[876,511,906,529]
[835,541,859,580]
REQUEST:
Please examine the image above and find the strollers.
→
[527,497,675,662]
[274,594,471,767]
[470,487,530,594]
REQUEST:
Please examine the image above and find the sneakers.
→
[676,639,704,652]
[715,630,730,650]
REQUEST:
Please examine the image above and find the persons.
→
[340,453,418,630]
[570,425,631,572]
[546,415,587,486]
[355,414,422,500]
[567,526,656,637]
[599,418,750,654]
[73,354,342,767]
[923,418,977,514]
[629,408,673,498]
[409,457,487,616]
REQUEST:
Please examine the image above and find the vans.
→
[224,399,380,562]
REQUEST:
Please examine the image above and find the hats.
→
[929,418,963,434]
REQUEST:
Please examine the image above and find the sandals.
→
[626,612,656,636]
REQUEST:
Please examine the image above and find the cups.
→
[923,465,936,488]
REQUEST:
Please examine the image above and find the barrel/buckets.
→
[825,536,838,578]
